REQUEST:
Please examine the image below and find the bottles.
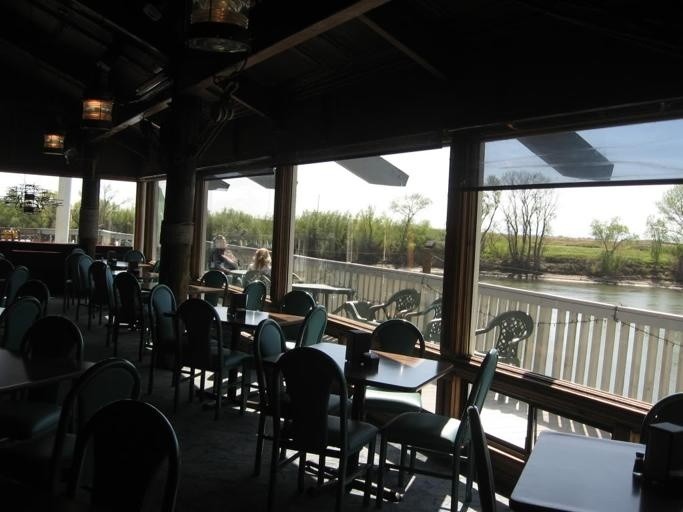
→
[632,451,645,477]
[226,304,234,320]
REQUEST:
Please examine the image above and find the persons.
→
[211,231,243,288]
[246,247,272,280]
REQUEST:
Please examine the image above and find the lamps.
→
[77,87,116,131]
[42,128,67,157]
[183,0,254,55]
